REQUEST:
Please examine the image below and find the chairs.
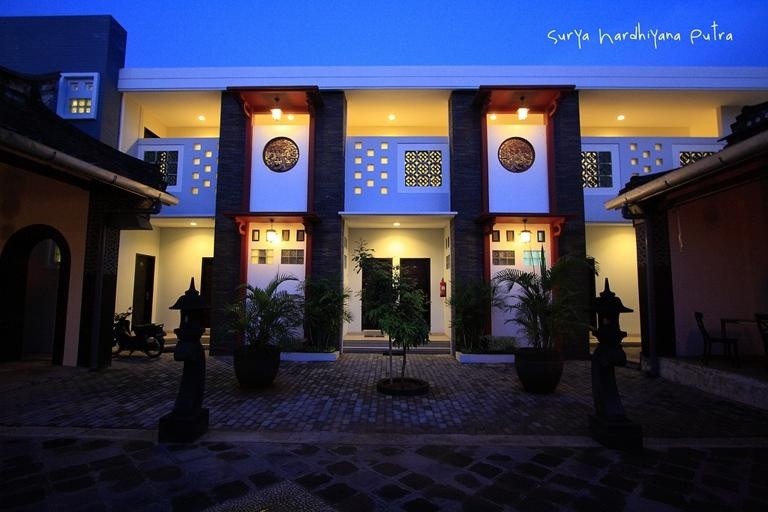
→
[695,311,739,365]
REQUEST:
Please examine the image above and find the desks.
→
[721,317,761,361]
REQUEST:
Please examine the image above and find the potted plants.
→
[484,247,597,393]
[212,276,304,390]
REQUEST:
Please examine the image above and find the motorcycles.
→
[112,305,167,358]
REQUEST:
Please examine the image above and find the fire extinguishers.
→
[440,278,446,297]
